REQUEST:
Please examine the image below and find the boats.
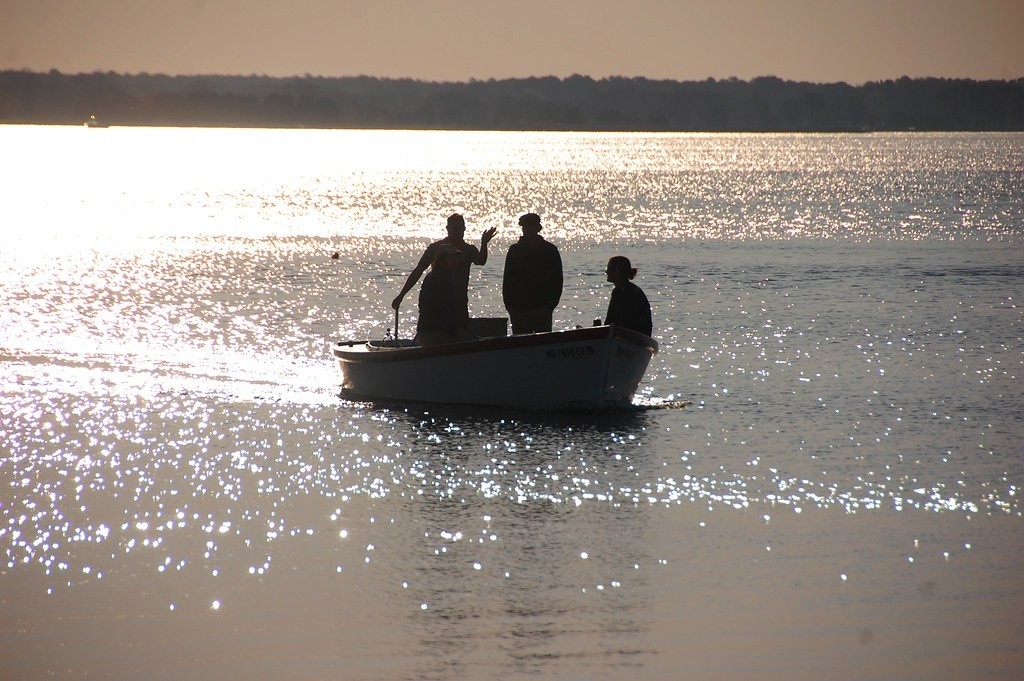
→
[87,116,112,128]
[333,309,659,415]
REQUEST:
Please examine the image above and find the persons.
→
[604,256,654,338]
[502,213,564,335]
[391,213,498,346]
